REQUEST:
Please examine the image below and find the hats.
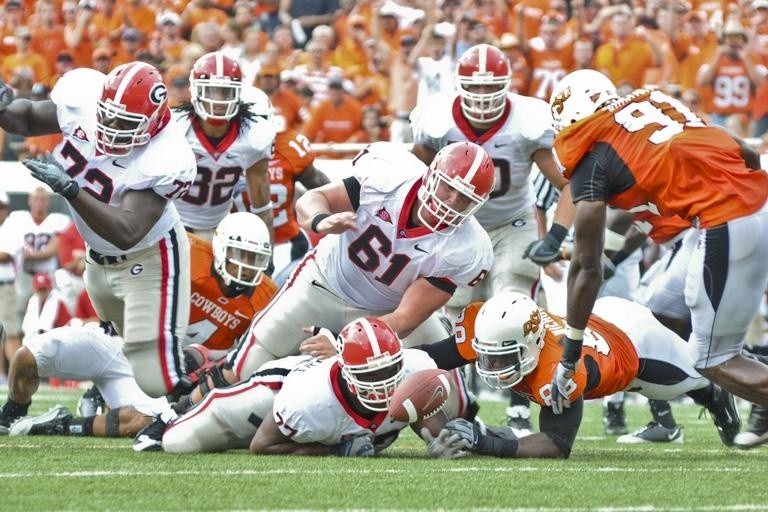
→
[668,1,768,43]
[5,1,205,61]
[33,271,51,290]
[259,64,281,74]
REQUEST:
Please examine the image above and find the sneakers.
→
[0,394,105,435]
[132,412,167,452]
[601,400,628,435]
[694,349,768,449]
[616,421,685,443]
[507,415,537,435]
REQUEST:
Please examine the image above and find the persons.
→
[2,1,768,457]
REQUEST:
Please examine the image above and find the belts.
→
[90,249,127,265]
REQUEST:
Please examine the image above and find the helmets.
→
[470,291,546,391]
[189,51,243,125]
[212,211,272,287]
[549,69,620,135]
[416,142,496,237]
[336,317,407,413]
[239,86,274,119]
[92,61,171,157]
[455,43,512,125]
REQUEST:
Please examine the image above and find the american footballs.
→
[386,368,454,422]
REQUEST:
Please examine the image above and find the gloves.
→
[0,79,13,111]
[338,433,374,457]
[550,356,582,415]
[22,149,79,201]
[522,223,568,266]
[420,418,482,459]
